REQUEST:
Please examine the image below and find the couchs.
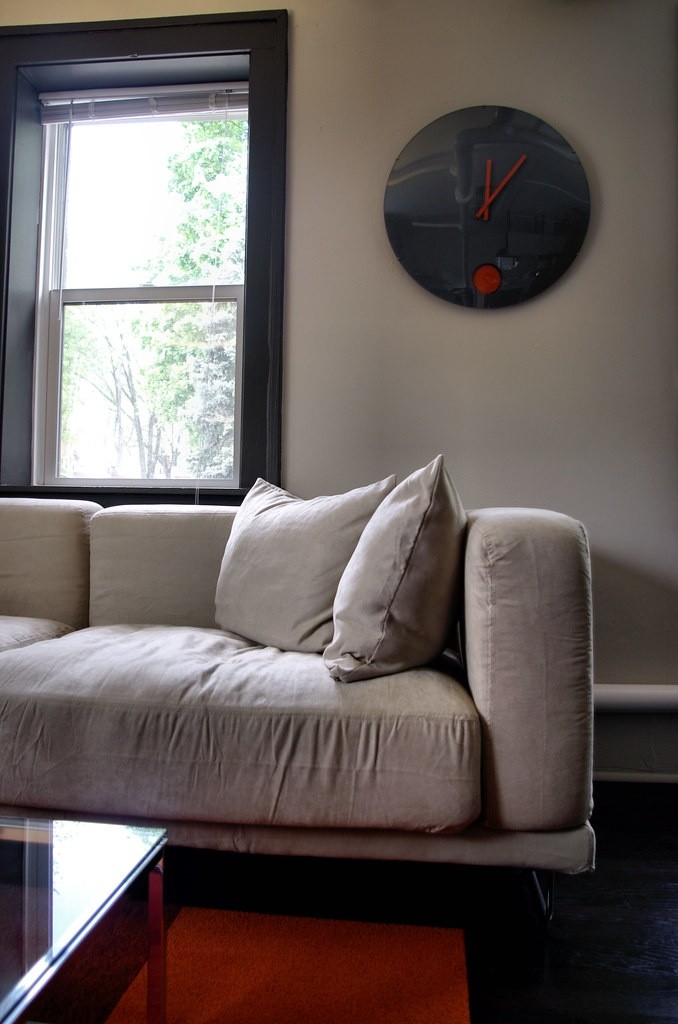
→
[0,498,597,929]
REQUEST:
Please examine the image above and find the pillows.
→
[212,475,396,656]
[324,454,467,684]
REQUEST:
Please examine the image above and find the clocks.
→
[382,105,594,314]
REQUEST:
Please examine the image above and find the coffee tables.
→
[0,812,173,1024]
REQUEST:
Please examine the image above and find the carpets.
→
[105,904,472,1024]
